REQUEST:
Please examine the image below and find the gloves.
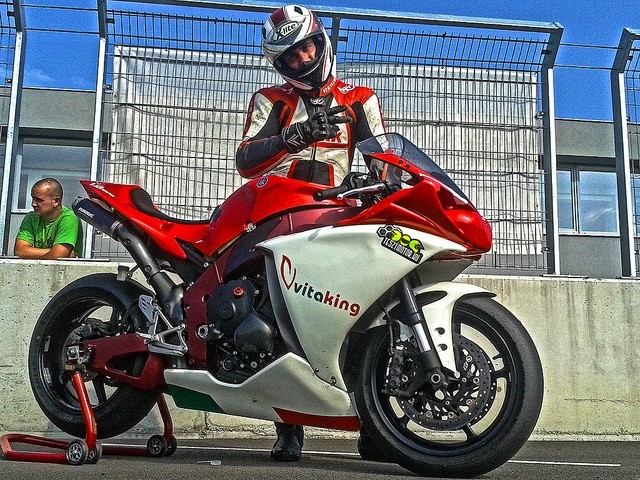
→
[281,105,353,154]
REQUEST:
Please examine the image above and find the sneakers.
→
[357,427,397,462]
[270,421,304,462]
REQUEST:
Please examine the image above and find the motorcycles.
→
[0,132,544,478]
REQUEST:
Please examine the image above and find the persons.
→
[234,4,403,463]
[13,177,83,260]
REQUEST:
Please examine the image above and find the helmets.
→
[261,5,334,91]
